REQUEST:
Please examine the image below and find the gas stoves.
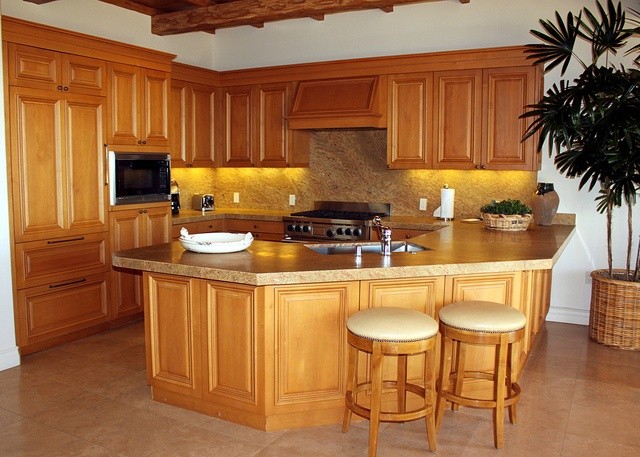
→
[282,209,392,241]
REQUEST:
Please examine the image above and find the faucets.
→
[371,215,392,256]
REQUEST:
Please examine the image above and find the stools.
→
[341,307,439,457]
[434,301,527,448]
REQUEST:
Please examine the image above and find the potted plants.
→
[480,199,534,232]
[517,0,640,351]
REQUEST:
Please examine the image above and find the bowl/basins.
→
[180,228,253,253]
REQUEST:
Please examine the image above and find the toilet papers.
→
[432,187,456,219]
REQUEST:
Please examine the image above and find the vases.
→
[531,183,559,226]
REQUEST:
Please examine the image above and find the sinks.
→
[302,240,432,255]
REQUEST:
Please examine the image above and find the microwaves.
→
[107,150,171,206]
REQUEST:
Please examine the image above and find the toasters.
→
[191,193,214,211]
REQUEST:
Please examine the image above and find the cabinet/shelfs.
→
[387,52,433,170]
[15,232,111,355]
[172,218,226,240]
[226,218,284,242]
[109,202,173,328]
[171,60,220,168]
[221,65,309,167]
[432,44,544,171]
[8,16,107,97]
[8,85,109,243]
[370,226,433,242]
[106,39,177,152]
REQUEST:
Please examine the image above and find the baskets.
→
[481,212,533,231]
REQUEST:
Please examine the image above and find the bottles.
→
[170,178,180,217]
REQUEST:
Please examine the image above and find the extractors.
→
[287,81,385,133]
